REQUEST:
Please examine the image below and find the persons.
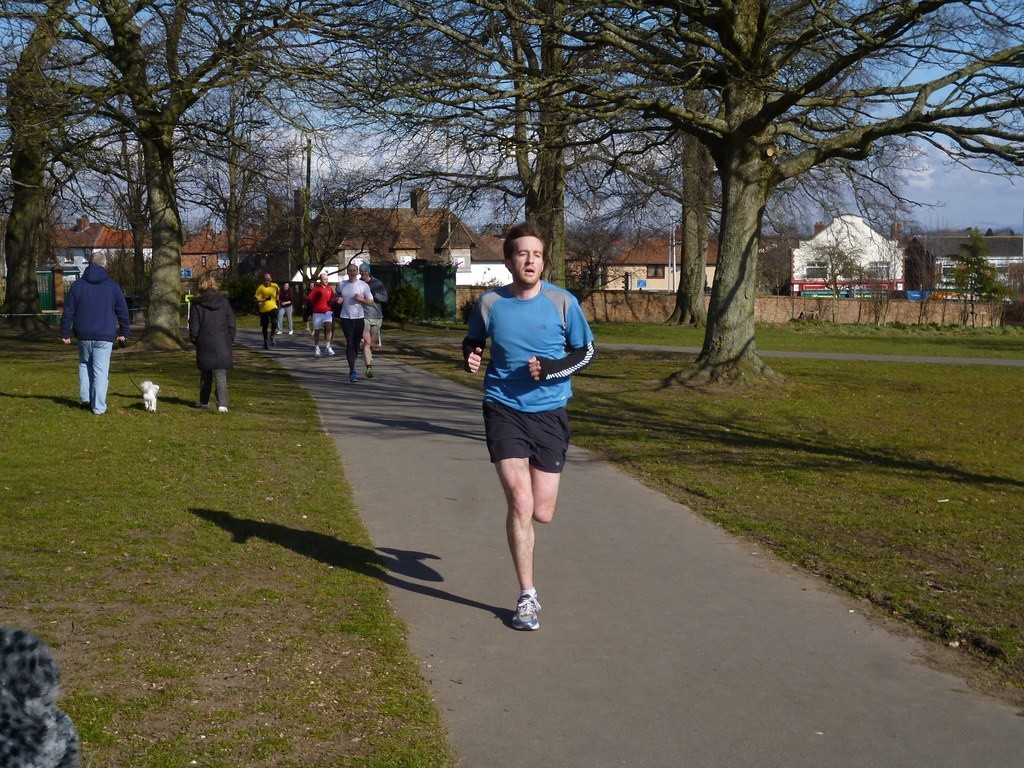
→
[59,252,130,415]
[302,273,336,358]
[335,264,374,382]
[462,220,596,631]
[188,275,237,412]
[359,263,388,378]
[254,273,294,349]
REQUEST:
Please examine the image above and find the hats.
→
[359,263,370,274]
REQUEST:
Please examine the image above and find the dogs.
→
[140,381,159,413]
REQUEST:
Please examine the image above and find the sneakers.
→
[365,366,372,378]
[324,346,336,356]
[511,590,542,631]
[349,371,358,383]
[315,346,322,358]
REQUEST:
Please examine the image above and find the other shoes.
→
[288,330,294,335]
[263,340,268,349]
[276,331,283,335]
[217,405,229,413]
[195,401,210,409]
[269,335,276,346]
